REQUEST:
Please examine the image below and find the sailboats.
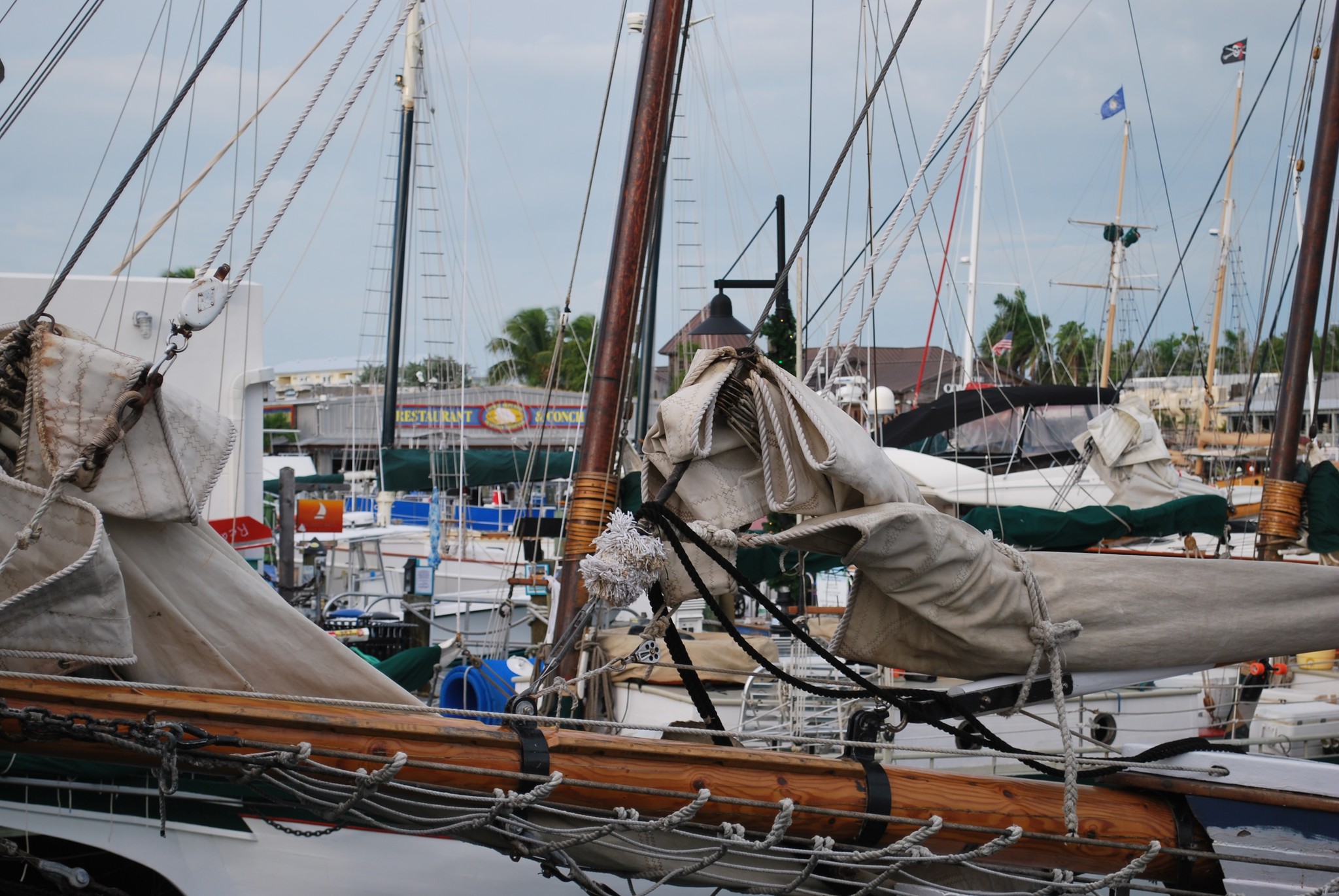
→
[0,0,1339,896]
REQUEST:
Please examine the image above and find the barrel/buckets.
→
[493,490,503,505]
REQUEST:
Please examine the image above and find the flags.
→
[1100,87,1125,120]
[1220,38,1246,65]
[991,330,1014,357]
